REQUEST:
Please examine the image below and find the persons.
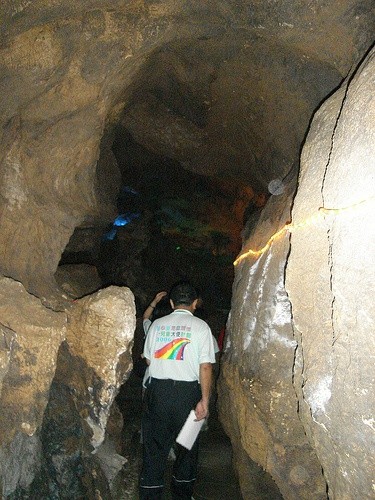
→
[139,282,216,500]
[143,291,221,459]
